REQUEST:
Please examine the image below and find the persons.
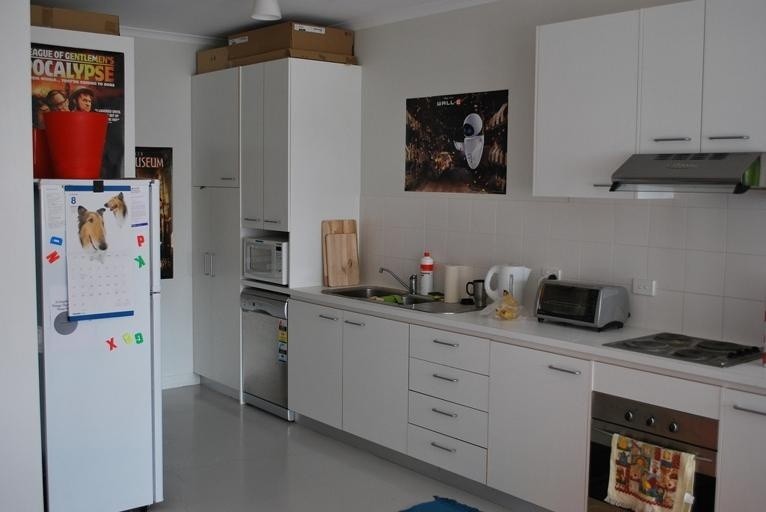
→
[33,100,50,127]
[70,85,94,112]
[45,90,70,111]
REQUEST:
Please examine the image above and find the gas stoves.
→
[603,330,764,367]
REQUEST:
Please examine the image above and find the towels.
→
[603,432,698,511]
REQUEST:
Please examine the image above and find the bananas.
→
[495,290,519,320]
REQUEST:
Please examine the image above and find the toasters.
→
[532,277,629,330]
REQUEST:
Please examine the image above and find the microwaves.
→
[243,238,287,285]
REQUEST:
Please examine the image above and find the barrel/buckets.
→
[43,110,109,179]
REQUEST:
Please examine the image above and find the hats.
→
[69,85,95,101]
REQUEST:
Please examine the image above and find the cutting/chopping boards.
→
[320,219,358,285]
[325,232,360,287]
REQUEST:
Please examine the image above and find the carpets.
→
[399,494,485,511]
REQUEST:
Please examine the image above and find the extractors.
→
[610,150,761,193]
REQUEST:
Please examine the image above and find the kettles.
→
[484,265,531,306]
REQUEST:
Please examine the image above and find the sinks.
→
[322,285,409,300]
[371,294,436,307]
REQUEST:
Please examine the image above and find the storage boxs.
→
[226,21,359,68]
[30,5,119,35]
[195,47,227,75]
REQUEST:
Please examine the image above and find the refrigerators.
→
[32,175,166,512]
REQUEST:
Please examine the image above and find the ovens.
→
[585,390,720,512]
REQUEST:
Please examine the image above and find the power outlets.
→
[541,266,561,280]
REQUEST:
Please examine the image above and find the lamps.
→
[251,1,283,21]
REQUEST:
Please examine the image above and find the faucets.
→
[379,267,417,295]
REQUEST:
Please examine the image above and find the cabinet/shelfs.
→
[189,65,240,188]
[487,338,591,511]
[289,297,407,465]
[190,188,242,392]
[636,1,766,155]
[240,58,360,230]
[716,385,766,511]
[407,320,488,488]
[533,4,674,201]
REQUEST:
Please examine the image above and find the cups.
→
[467,279,487,307]
